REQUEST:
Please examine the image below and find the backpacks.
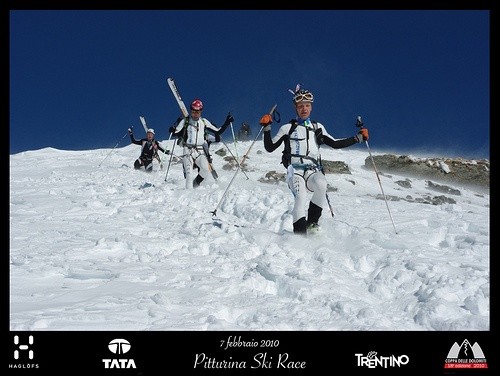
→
[177,115,207,147]
[282,119,322,168]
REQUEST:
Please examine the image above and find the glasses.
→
[294,93,313,102]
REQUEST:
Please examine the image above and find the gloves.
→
[227,115,234,122]
[359,129,368,141]
[260,115,272,126]
[128,127,132,134]
[170,126,176,132]
[165,150,170,155]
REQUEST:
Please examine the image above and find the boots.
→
[307,200,322,230]
[193,174,204,188]
[293,217,307,238]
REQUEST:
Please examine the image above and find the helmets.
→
[148,129,155,134]
[191,100,203,111]
[293,90,315,104]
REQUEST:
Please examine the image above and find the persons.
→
[259,88,369,235]
[127,127,171,172]
[168,98,234,190]
[176,121,221,179]
